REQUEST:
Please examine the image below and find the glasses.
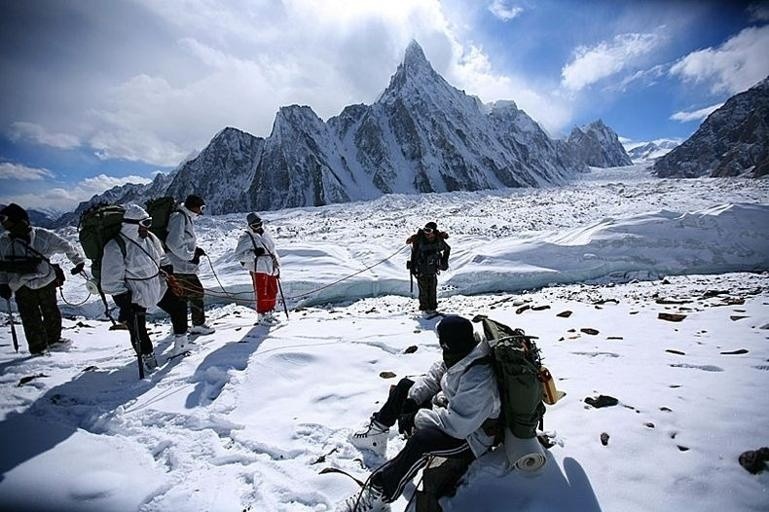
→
[249,221,264,230]
[199,204,207,213]
[0,214,9,224]
[138,216,153,229]
[423,227,434,234]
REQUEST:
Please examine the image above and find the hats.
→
[435,316,473,340]
[425,222,437,230]
[0,203,30,224]
[184,195,205,209]
[246,212,261,225]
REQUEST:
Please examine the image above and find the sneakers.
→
[165,334,190,361]
[256,310,281,327]
[29,336,74,360]
[333,481,392,512]
[421,306,437,320]
[141,351,159,373]
[189,322,215,335]
[347,416,389,457]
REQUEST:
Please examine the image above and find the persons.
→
[234,209,282,326]
[100,203,189,377]
[160,194,217,361]
[335,314,510,511]
[404,220,451,321]
[0,201,86,362]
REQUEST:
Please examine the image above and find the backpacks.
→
[472,315,546,439]
[77,203,126,280]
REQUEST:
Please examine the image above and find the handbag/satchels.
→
[49,263,66,287]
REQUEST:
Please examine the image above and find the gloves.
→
[188,247,205,266]
[112,290,143,323]
[0,283,12,300]
[253,247,265,258]
[159,265,174,278]
[398,398,420,440]
